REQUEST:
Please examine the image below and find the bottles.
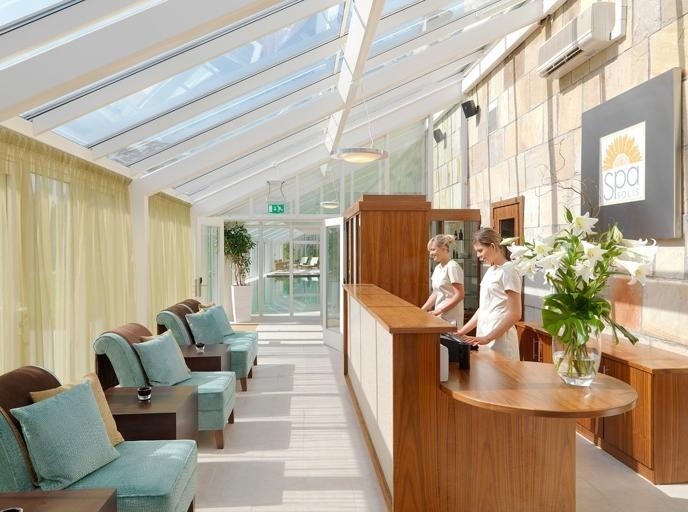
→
[456,251,458,258]
[459,229,463,240]
[454,230,457,240]
[453,249,456,258]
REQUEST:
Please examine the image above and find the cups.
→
[459,341,470,369]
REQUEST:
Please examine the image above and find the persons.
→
[448,228,523,358]
[421,234,464,328]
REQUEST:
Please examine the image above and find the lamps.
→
[337,81,388,164]
[320,171,339,209]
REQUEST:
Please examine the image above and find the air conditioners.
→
[538,1,615,81]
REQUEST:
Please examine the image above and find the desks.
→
[179,343,230,371]
[103,385,198,448]
[0,488,118,512]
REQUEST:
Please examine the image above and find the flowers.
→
[500,206,658,377]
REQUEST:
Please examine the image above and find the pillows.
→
[185,312,224,348]
[29,371,125,447]
[132,330,192,387]
[140,329,191,374]
[9,379,120,491]
[198,303,216,311]
[205,306,234,337]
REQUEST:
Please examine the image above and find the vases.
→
[552,320,601,387]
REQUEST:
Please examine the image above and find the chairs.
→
[294,257,308,269]
[302,257,319,269]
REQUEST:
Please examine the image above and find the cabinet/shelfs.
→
[344,195,481,377]
[516,326,553,363]
[576,353,688,485]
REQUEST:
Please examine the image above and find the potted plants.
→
[224,220,256,323]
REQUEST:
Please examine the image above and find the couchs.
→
[157,299,259,391]
[1,366,198,512]
[94,323,236,449]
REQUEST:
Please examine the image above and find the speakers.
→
[433,128,444,144]
[461,100,478,118]
